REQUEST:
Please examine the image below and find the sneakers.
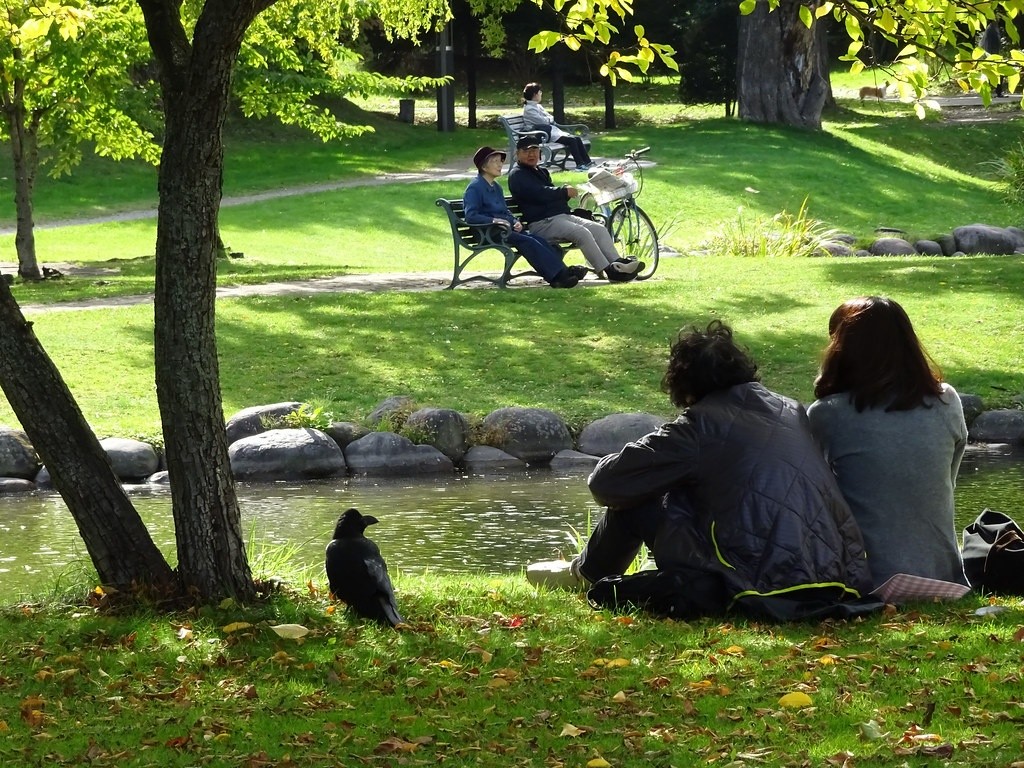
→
[526,560,585,595]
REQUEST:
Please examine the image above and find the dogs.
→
[860,80,891,107]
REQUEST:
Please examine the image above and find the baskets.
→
[588,164,639,206]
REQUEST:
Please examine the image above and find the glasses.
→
[539,91,542,94]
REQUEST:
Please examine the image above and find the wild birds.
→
[324,507,404,629]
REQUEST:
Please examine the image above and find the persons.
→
[506,136,646,285]
[461,147,589,289]
[520,82,597,171]
[801,293,973,594]
[526,318,872,621]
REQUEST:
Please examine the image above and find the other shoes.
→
[576,162,597,172]
[608,258,646,281]
[564,265,588,278]
[550,276,578,288]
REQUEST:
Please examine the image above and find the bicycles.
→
[576,145,663,281]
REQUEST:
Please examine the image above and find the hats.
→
[472,146,507,168]
[517,136,541,150]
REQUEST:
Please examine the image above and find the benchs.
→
[436,195,610,290]
[498,113,591,175]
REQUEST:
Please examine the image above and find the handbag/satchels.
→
[520,124,552,143]
[963,508,1024,593]
[574,209,592,220]
[587,565,728,622]
